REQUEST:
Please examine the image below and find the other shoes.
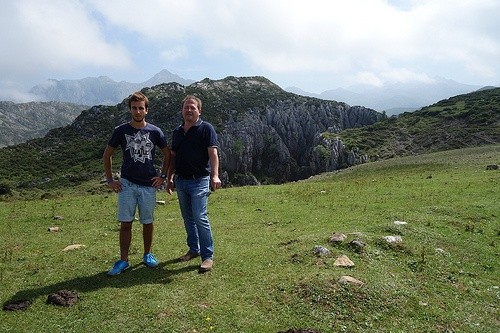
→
[178,251,197,260]
[200,259,213,270]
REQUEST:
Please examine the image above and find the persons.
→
[165,94,222,272]
[103,91,173,276]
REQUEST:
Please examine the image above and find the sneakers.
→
[144,253,158,267]
[107,260,130,276]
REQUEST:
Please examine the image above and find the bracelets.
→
[106,177,114,184]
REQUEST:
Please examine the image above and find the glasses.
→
[206,187,214,197]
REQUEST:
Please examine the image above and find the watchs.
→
[159,171,167,179]
